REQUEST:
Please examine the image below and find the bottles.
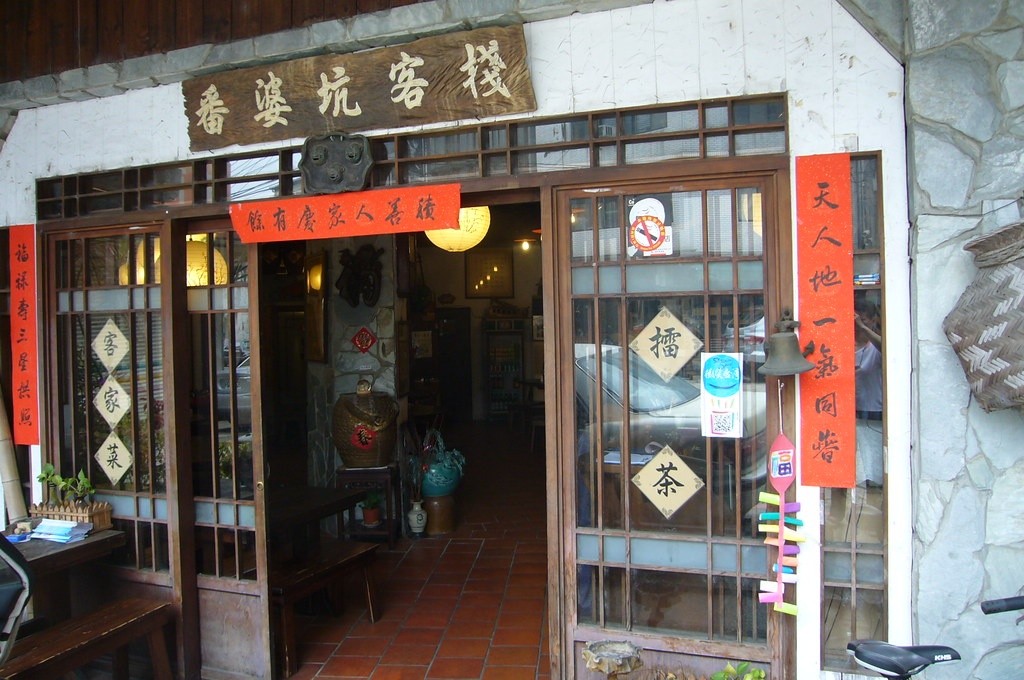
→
[539,375,544,401]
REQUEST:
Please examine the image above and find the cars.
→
[719,305,770,383]
[571,339,779,612]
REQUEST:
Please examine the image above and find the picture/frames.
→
[464,249,514,300]
[532,315,544,341]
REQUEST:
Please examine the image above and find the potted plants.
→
[28,463,112,535]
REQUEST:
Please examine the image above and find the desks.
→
[198,488,368,575]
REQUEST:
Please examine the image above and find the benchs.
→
[0,595,173,680]
[255,538,384,680]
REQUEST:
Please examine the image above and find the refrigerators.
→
[483,317,524,425]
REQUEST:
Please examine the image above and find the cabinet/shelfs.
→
[337,462,401,550]
[482,314,525,423]
[0,528,126,633]
[407,308,472,435]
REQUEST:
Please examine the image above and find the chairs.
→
[529,419,545,453]
[509,382,546,433]
